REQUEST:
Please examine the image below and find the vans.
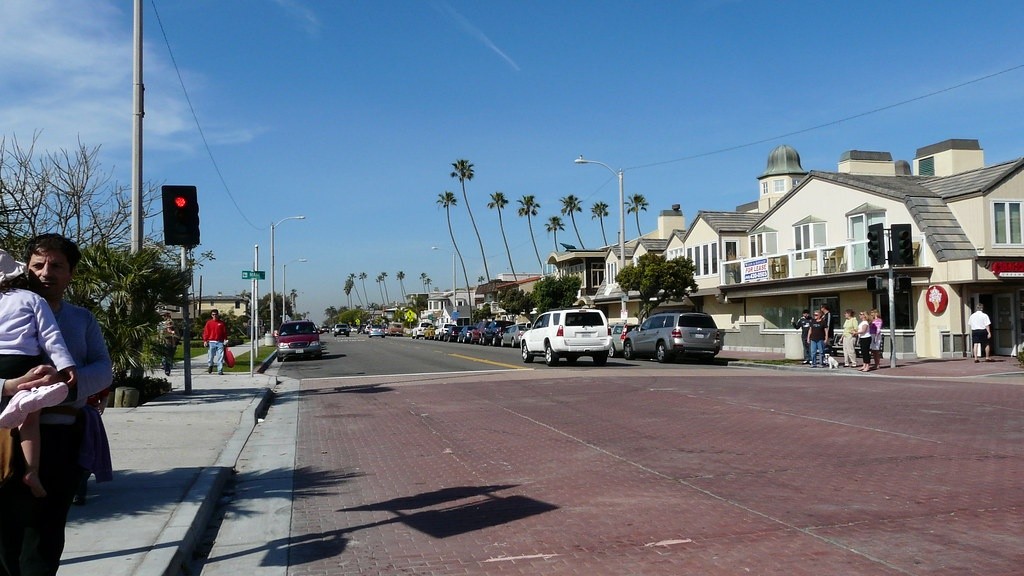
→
[274,320,324,362]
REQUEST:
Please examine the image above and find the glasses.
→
[212,314,217,316]
[814,314,818,316]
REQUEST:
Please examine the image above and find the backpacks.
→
[869,322,877,334]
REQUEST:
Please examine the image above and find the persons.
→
[791,303,882,372]
[203,309,227,375]
[0,234,112,576]
[0,250,79,497]
[969,303,995,363]
[161,313,180,376]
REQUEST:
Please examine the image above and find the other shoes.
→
[165,367,170,375]
[986,358,995,362]
[802,361,880,372]
[975,360,980,363]
[208,367,212,373]
[219,373,223,375]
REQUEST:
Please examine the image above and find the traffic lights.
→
[891,224,913,265]
[162,185,201,247]
[867,276,882,292]
[867,223,885,266]
[895,276,912,294]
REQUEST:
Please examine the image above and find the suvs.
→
[622,310,720,364]
[607,322,641,358]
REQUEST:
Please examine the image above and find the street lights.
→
[431,246,457,307]
[270,216,305,334]
[282,259,308,322]
[574,158,627,324]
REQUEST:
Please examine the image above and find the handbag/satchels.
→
[224,345,235,368]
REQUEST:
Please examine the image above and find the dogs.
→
[824,354,839,369]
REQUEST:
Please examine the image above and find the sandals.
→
[0,390,32,428]
[19,381,68,412]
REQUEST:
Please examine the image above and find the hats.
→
[0,249,26,282]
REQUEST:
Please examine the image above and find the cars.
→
[351,324,404,339]
[410,321,530,348]
[520,309,613,369]
[321,325,329,333]
[334,324,350,337]
